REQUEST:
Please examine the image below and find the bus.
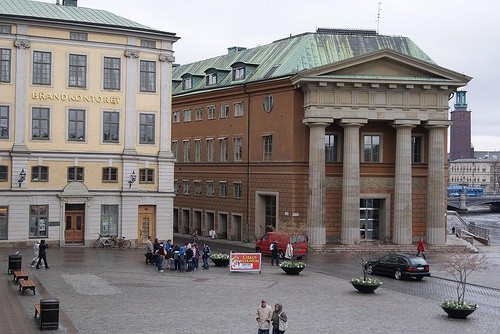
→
[447,186,485,197]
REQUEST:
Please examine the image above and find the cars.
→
[362,253,432,280]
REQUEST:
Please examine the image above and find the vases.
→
[210,259,230,268]
[280,266,306,276]
[438,302,476,319]
[349,281,380,294]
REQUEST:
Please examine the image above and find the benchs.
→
[34,304,41,329]
[19,280,36,296]
[13,271,28,285]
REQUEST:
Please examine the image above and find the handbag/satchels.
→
[279,318,288,331]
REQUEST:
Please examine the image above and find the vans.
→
[255,232,308,260]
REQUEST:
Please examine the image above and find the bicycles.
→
[93,233,132,249]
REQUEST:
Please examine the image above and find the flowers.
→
[209,252,229,260]
[278,259,307,268]
[441,297,479,310]
[351,276,383,287]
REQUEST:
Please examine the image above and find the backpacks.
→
[186,248,193,259]
[179,254,186,263]
[270,244,274,250]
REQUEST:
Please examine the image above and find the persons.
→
[30,240,45,268]
[452,227,455,233]
[285,243,293,262]
[145,236,199,272]
[271,240,279,266]
[36,240,50,269]
[202,244,210,269]
[271,303,287,334]
[416,238,425,259]
[256,299,272,334]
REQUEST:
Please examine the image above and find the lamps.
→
[18,169,26,187]
[128,170,137,188]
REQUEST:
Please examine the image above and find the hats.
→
[274,241,278,244]
[261,298,267,302]
[37,240,41,243]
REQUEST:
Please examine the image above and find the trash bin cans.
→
[8,254,21,274]
[40,298,59,330]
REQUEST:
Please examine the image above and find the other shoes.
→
[159,269,164,273]
[36,266,40,269]
[41,264,45,267]
[46,266,51,269]
[32,265,34,268]
